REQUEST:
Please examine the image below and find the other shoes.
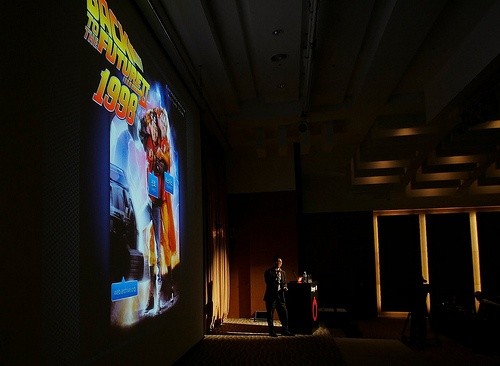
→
[280,328,291,336]
[269,330,277,337]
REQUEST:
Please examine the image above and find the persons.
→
[112,106,180,316]
[263,255,295,337]
